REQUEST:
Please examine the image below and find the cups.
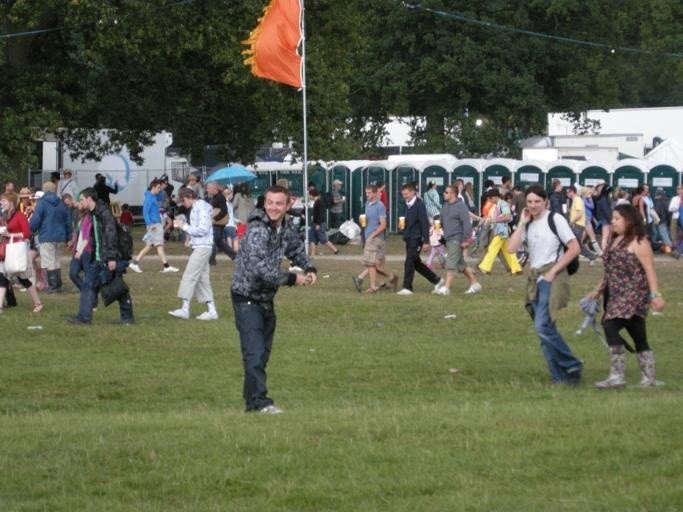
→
[358,214,367,227]
[398,216,405,230]
[434,220,440,231]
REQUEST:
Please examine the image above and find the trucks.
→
[28,126,195,217]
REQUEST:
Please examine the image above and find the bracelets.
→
[650,291,662,298]
[519,219,527,226]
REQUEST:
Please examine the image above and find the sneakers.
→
[2,263,218,324]
[567,370,580,387]
[261,405,283,414]
[352,264,523,296]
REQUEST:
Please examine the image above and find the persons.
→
[1,170,348,325]
[549,179,683,259]
[354,179,526,297]
[505,185,585,387]
[587,204,667,389]
[230,184,317,414]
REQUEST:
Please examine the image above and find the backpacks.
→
[525,211,579,276]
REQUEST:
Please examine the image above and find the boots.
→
[629,351,655,388]
[594,346,626,389]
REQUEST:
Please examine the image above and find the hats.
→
[18,188,30,198]
[333,180,343,184]
[29,191,44,199]
[487,188,498,196]
[42,182,56,194]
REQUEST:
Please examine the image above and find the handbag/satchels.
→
[96,208,133,261]
[6,233,30,273]
[508,220,517,235]
